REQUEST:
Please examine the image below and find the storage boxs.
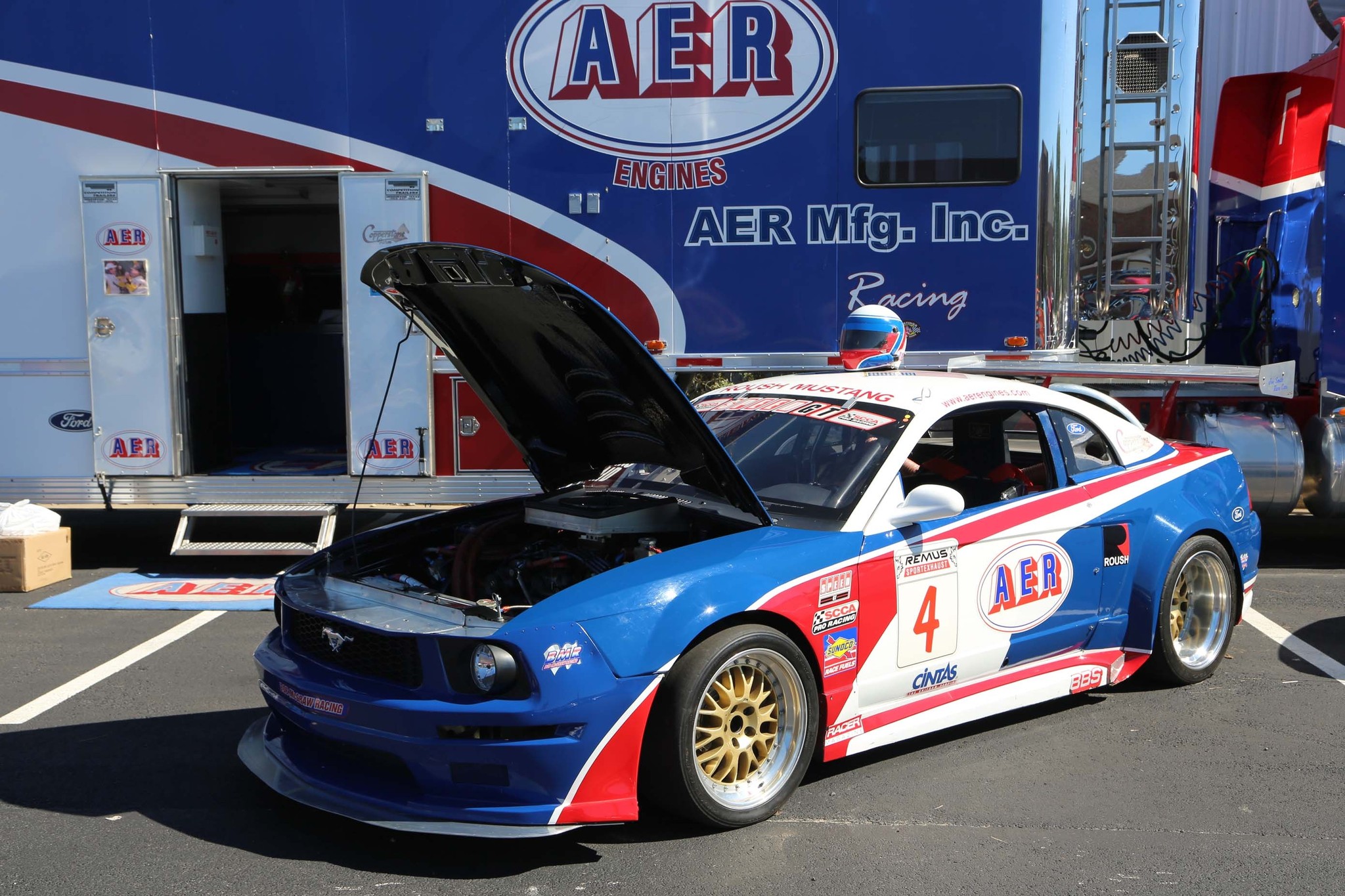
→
[0,526,72,593]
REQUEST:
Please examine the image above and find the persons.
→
[842,420,957,493]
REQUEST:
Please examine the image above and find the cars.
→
[235,242,1261,840]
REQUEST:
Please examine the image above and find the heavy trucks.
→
[0,1,1345,563]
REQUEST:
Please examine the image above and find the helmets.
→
[838,304,908,371]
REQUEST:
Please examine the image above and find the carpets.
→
[24,570,279,612]
